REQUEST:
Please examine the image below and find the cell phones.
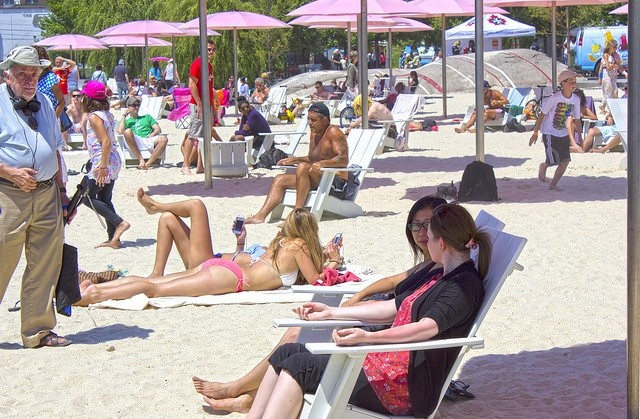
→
[233,216,245,235]
[334,233,342,244]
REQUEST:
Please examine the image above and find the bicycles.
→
[524,84,562,119]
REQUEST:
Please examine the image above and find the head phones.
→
[6,84,41,112]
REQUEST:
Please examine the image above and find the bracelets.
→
[100,167,107,169]
[330,260,339,265]
[59,187,67,193]
[122,114,126,117]
[533,129,538,136]
[236,243,245,246]
[498,100,501,107]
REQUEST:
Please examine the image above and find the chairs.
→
[371,97,386,101]
[617,78,628,98]
[167,87,193,129]
[290,210,506,343]
[256,84,288,125]
[115,108,169,169]
[352,94,427,155]
[581,120,608,149]
[138,94,163,122]
[198,138,249,187]
[372,76,397,90]
[457,83,507,130]
[472,87,532,131]
[108,78,124,100]
[251,104,315,163]
[215,89,231,118]
[402,76,423,94]
[298,84,335,118]
[271,128,390,222]
[164,82,186,111]
[229,78,248,104]
[585,96,596,115]
[606,98,628,154]
[271,225,528,418]
[66,132,91,151]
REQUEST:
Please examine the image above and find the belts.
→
[0,177,57,190]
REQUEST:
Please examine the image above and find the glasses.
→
[71,94,81,98]
[407,221,431,231]
[132,103,140,107]
[208,48,215,53]
[432,199,462,233]
[22,106,39,130]
[241,105,247,110]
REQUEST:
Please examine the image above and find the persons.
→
[337,50,359,111]
[181,41,215,175]
[238,77,250,102]
[529,68,582,193]
[570,87,628,155]
[292,80,329,117]
[368,51,385,69]
[164,83,180,110]
[408,71,418,93]
[376,82,404,111]
[345,94,399,141]
[435,49,442,61]
[62,89,87,153]
[1,47,77,348]
[164,59,181,90]
[149,62,164,82]
[80,80,130,249]
[29,46,69,187]
[137,187,269,278]
[129,80,146,95]
[245,102,349,228]
[332,49,341,71]
[135,87,158,100]
[601,43,617,116]
[453,81,509,133]
[464,40,475,55]
[331,79,338,87]
[452,40,462,55]
[244,203,494,418]
[236,95,245,103]
[192,195,448,414]
[224,75,236,92]
[570,87,598,134]
[252,77,270,102]
[181,120,223,166]
[406,52,420,69]
[562,42,569,65]
[118,96,169,172]
[409,120,433,133]
[71,207,342,307]
[52,55,77,104]
[229,101,271,159]
[92,63,107,86]
[114,59,132,101]
[598,39,628,84]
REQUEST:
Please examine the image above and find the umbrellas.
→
[99,35,174,77]
[308,25,358,30]
[166,20,222,74]
[30,33,106,61]
[348,17,434,71]
[45,46,110,71]
[288,17,398,66]
[608,4,629,16]
[490,1,624,95]
[405,1,511,117]
[285,0,432,128]
[94,19,189,90]
[179,10,292,99]
[148,57,171,83]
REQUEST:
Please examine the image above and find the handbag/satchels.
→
[261,149,288,168]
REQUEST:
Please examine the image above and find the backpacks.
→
[460,161,500,201]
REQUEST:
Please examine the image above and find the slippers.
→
[25,332,73,348]
[450,379,475,396]
[445,387,458,400]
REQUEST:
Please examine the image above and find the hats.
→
[0,46,53,72]
[80,82,107,100]
[125,97,137,105]
[558,71,578,83]
[310,103,329,116]
